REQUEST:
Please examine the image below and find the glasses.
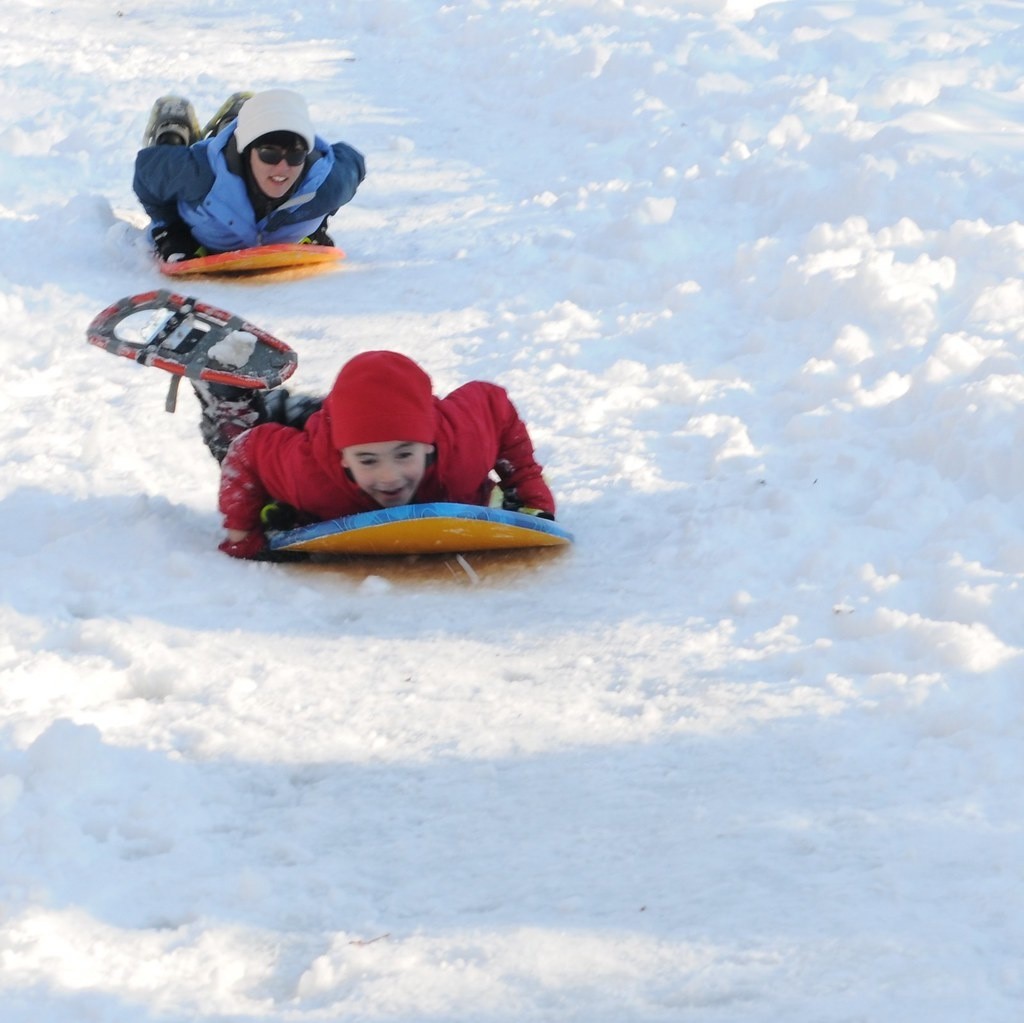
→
[254,146,309,166]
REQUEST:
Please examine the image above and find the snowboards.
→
[264,498,575,560]
[152,242,345,276]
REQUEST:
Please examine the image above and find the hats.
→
[234,88,315,155]
[326,351,438,449]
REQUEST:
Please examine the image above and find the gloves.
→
[298,223,336,247]
[152,218,206,263]
[220,531,308,562]
[518,505,555,524]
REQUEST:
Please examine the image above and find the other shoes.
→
[205,112,238,140]
[149,119,191,150]
[189,378,258,407]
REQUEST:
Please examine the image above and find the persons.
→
[190,351,556,564]
[133,88,366,263]
